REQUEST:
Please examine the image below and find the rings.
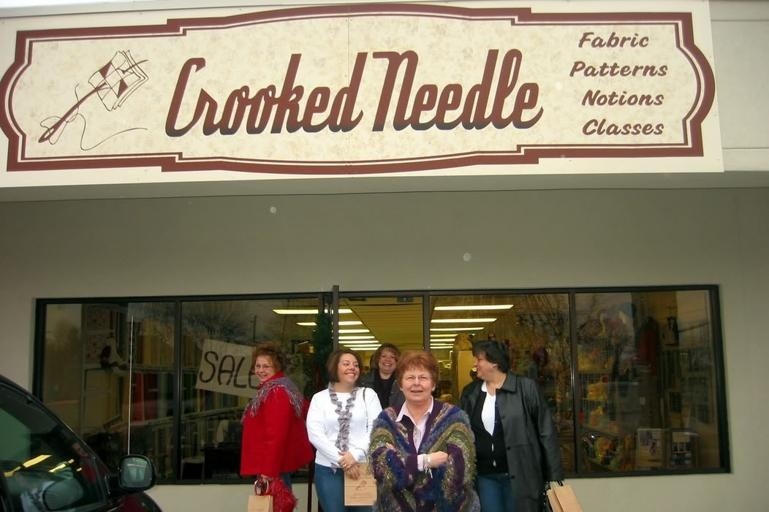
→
[343,464,347,467]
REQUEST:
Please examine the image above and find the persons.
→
[239,341,316,511]
[358,343,407,418]
[306,346,383,512]
[368,349,481,512]
[459,339,566,512]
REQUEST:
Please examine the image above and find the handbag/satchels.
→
[344,464,378,506]
[248,481,273,512]
[262,480,295,512]
[540,484,582,512]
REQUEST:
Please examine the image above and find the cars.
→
[0,375,163,510]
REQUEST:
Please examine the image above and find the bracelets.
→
[424,454,433,479]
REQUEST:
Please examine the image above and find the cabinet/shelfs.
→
[451,312,721,470]
[78,300,248,485]
